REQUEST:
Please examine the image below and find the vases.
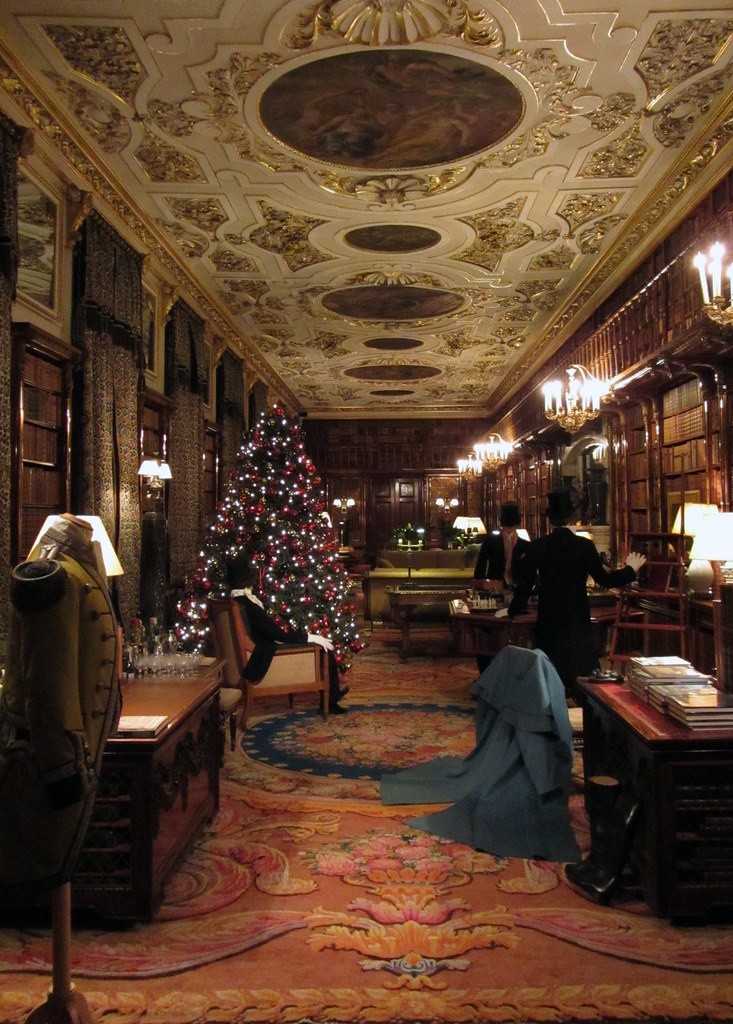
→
[587,469,608,525]
[558,476,578,511]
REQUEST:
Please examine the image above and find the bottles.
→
[585,467,608,525]
[466,584,539,608]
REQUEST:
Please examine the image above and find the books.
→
[624,380,723,534]
[627,656,733,731]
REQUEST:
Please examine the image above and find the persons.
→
[474,502,531,675]
[0,513,122,895]
[228,572,350,715]
[495,487,647,703]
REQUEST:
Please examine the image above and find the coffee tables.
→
[386,584,547,654]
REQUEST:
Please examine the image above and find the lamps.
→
[671,502,718,543]
[689,513,733,599]
[27,515,124,577]
[453,449,483,479]
[333,498,355,518]
[453,516,487,547]
[136,459,173,507]
[691,240,733,326]
[542,363,608,437]
[435,498,459,513]
[472,432,512,472]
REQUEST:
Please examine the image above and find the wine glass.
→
[132,653,203,682]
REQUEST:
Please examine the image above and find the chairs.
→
[214,682,241,751]
[203,598,329,728]
[502,643,620,804]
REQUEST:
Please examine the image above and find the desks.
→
[448,596,645,689]
[566,673,733,924]
[0,652,226,866]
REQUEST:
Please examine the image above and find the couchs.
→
[365,549,525,622]
